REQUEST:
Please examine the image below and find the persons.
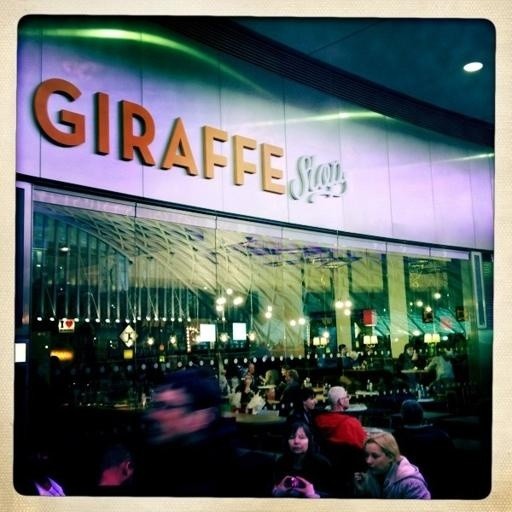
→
[93,342,456,499]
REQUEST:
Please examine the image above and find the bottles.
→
[402,399,423,424]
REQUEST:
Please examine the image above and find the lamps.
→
[237,366,470,431]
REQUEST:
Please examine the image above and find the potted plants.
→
[457,307,464,322]
[423,308,433,323]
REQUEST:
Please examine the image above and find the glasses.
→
[14,326,30,366]
[424,255,441,346]
[313,254,329,349]
[360,250,378,348]
